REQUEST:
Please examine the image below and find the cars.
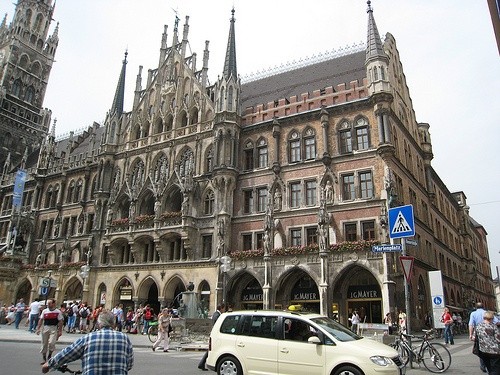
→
[205,304,405,375]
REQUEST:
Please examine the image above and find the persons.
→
[197,303,227,371]
[384,313,393,334]
[273,187,281,211]
[348,311,361,334]
[441,307,454,344]
[0,297,25,329]
[323,180,334,203]
[28,298,42,333]
[60,300,183,335]
[399,310,407,335]
[468,302,500,375]
[42,310,134,375]
[35,299,64,365]
[151,308,170,352]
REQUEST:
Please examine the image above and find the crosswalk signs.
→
[387,203,416,240]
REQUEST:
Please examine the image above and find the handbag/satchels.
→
[473,332,480,354]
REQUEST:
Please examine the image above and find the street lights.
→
[79,264,91,302]
[219,252,232,312]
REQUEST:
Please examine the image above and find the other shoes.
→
[40,360,47,365]
[164,350,168,352]
[152,346,155,351]
[127,331,130,333]
[480,366,486,372]
[198,366,208,371]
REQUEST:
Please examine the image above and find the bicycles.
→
[147,325,159,343]
[40,361,83,375]
[388,325,451,373]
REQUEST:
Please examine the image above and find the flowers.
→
[158,211,181,219]
[111,217,128,226]
[230,250,262,258]
[271,244,320,256]
[132,214,154,224]
[329,240,379,252]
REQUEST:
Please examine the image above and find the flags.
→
[13,169,27,207]
